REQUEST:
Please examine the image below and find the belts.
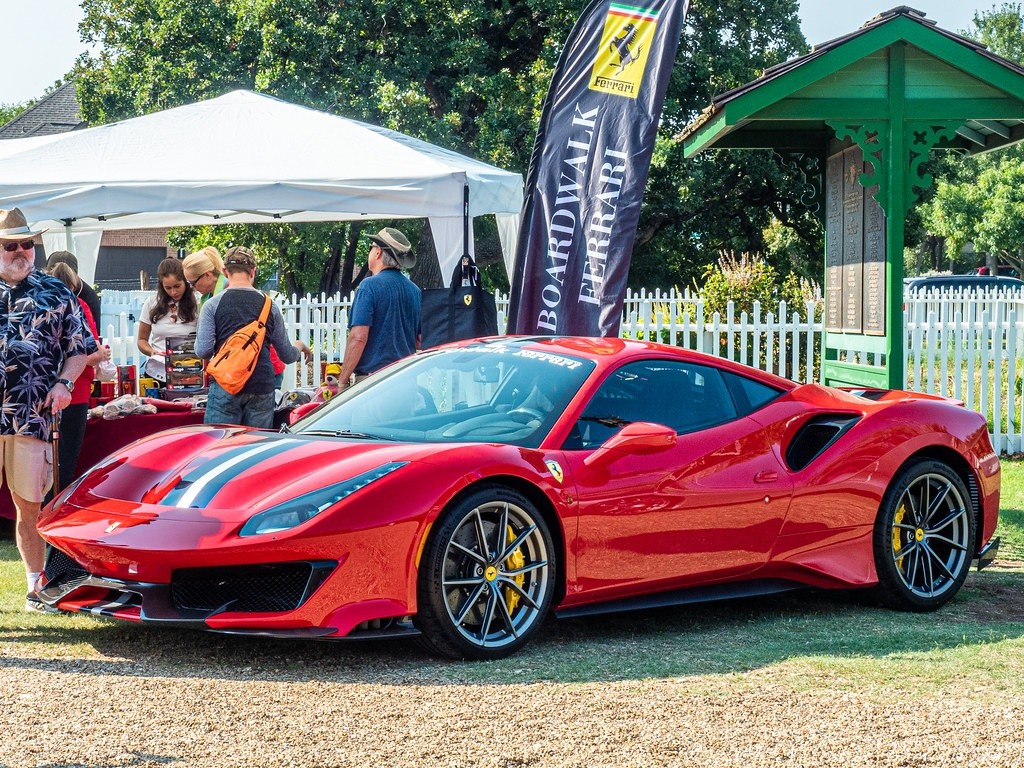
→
[354,372,368,377]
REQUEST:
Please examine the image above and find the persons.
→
[0,208,99,615]
[46,250,101,338]
[194,246,312,429]
[182,246,286,390]
[39,262,111,511]
[337,226,422,425]
[137,256,198,389]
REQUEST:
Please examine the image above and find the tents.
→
[0,89,525,405]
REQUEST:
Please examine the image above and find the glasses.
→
[169,302,177,323]
[187,274,203,286]
[0,239,35,251]
[369,242,381,251]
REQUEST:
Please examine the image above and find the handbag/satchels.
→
[419,254,499,351]
[206,292,272,395]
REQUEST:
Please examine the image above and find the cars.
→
[969,266,1021,279]
[910,275,1024,322]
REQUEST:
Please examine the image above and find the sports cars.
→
[171,358,202,366]
[172,376,202,383]
[197,399,208,407]
[35,336,1002,663]
[172,342,194,351]
[173,395,193,402]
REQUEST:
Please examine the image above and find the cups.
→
[145,389,161,399]
[139,378,160,397]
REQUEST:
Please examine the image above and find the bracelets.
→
[337,380,349,387]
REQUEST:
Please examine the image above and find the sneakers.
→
[25,595,64,615]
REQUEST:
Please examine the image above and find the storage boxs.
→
[165,335,210,390]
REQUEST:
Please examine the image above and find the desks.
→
[0,408,289,522]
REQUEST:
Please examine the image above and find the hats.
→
[0,207,49,239]
[366,226,417,268]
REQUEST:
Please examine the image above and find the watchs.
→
[57,378,75,392]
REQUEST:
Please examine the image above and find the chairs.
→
[513,378,565,418]
[646,370,694,428]
